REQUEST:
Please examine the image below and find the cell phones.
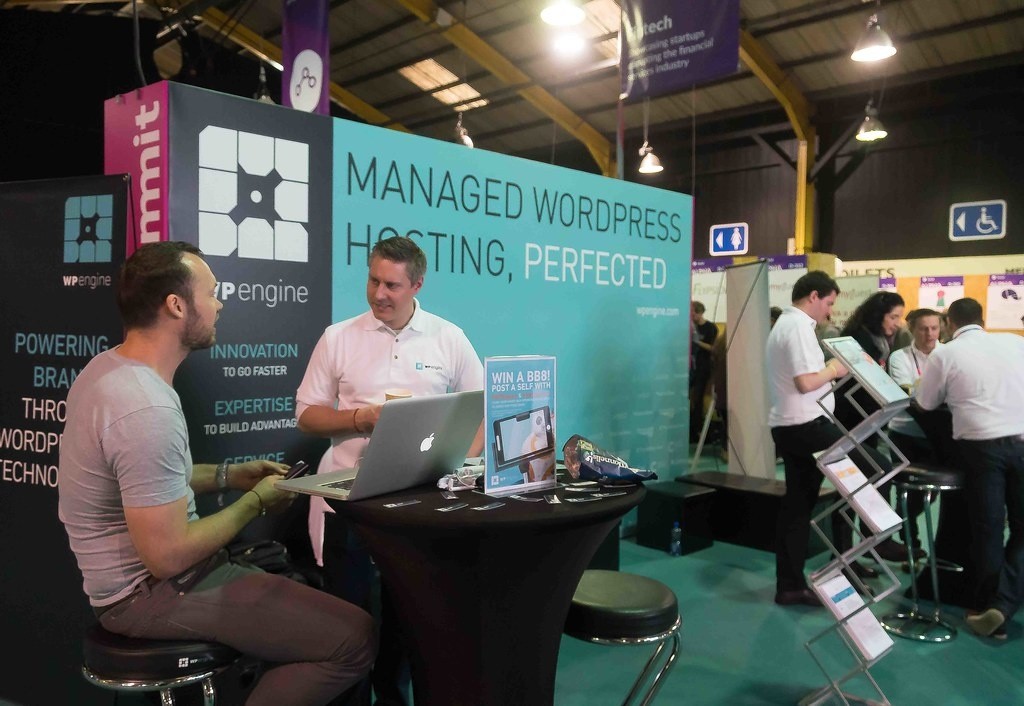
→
[284,462,309,481]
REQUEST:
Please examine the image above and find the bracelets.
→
[249,489,267,519]
[353,408,364,434]
[215,463,230,494]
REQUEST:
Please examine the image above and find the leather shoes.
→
[775,588,822,606]
[861,538,926,561]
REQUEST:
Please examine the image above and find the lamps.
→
[450,0,474,149]
[848,0,896,63]
[855,56,887,142]
[639,100,665,175]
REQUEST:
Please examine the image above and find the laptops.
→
[274,390,486,501]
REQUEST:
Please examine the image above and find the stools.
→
[873,456,966,642]
[562,569,683,706]
[76,624,241,706]
[636,481,716,556]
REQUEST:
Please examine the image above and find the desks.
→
[327,460,647,706]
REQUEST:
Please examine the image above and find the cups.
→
[386,388,412,400]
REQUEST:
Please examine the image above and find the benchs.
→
[679,469,841,557]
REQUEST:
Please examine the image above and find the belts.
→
[91,574,161,618]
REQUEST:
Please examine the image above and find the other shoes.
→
[965,609,1008,639]
[858,568,878,576]
[903,561,920,573]
[855,583,874,594]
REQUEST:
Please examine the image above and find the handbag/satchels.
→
[228,540,293,578]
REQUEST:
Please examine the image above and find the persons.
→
[58,241,378,706]
[295,237,486,706]
[770,290,1024,639]
[762,271,850,606]
[689,300,730,462]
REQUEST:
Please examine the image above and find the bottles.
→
[672,522,682,557]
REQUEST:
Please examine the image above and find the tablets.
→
[492,406,554,467]
